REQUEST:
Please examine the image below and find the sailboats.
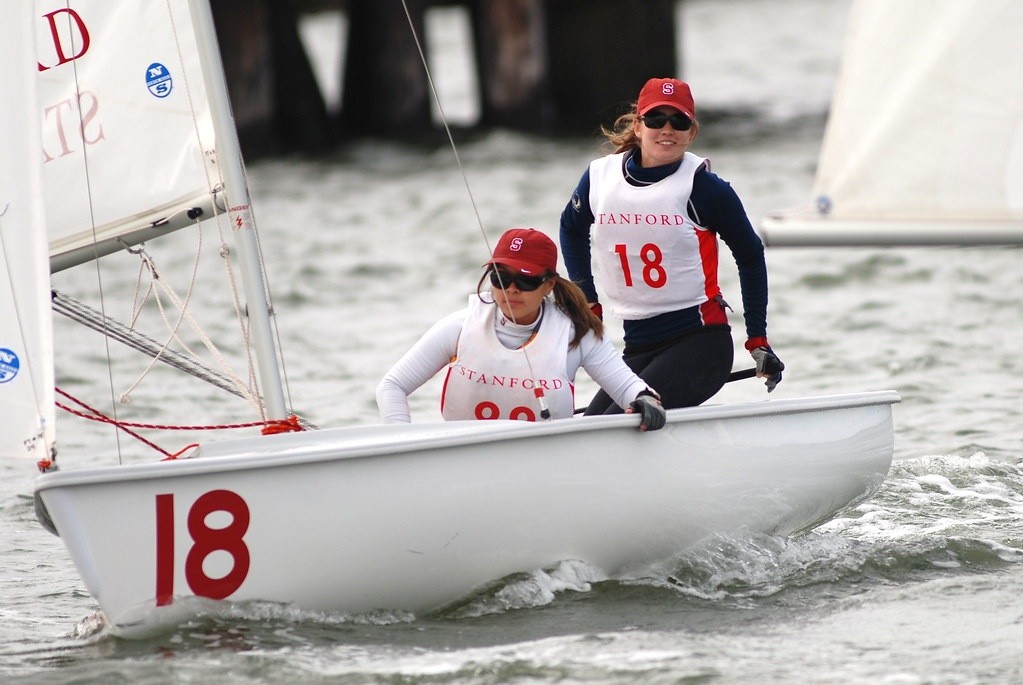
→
[1,1,901,640]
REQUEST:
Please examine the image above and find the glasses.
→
[490,270,553,292]
[638,110,694,131]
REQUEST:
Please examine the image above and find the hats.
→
[481,227,557,274]
[637,78,696,121]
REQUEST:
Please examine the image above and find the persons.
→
[377,228,666,432]
[560,78,782,416]
